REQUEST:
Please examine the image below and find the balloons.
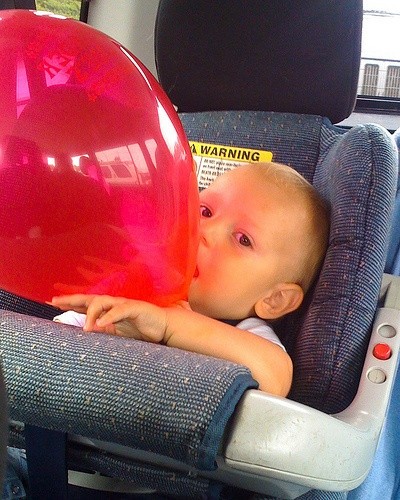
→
[0,9,200,306]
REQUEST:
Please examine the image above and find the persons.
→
[49,155,327,402]
